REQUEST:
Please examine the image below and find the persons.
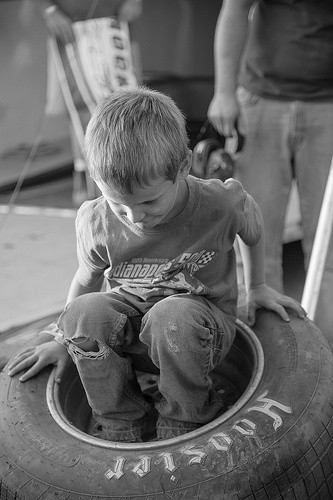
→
[7,86,308,445]
[38,0,143,205]
[207,0,333,343]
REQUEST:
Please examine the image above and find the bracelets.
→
[42,5,58,18]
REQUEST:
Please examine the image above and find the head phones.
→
[194,116,245,181]
